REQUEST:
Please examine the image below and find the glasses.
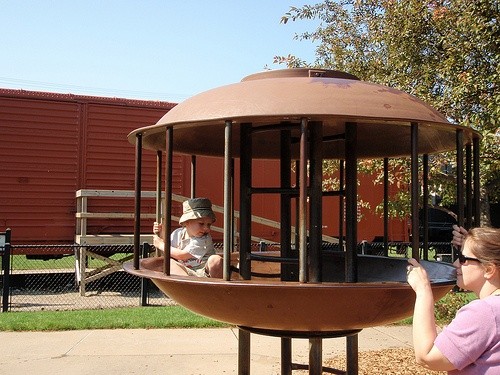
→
[452,249,483,265]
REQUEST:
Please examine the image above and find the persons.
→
[408,196,424,241]
[405,224,500,375]
[153,197,223,279]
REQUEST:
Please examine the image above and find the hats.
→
[178,197,215,227]
